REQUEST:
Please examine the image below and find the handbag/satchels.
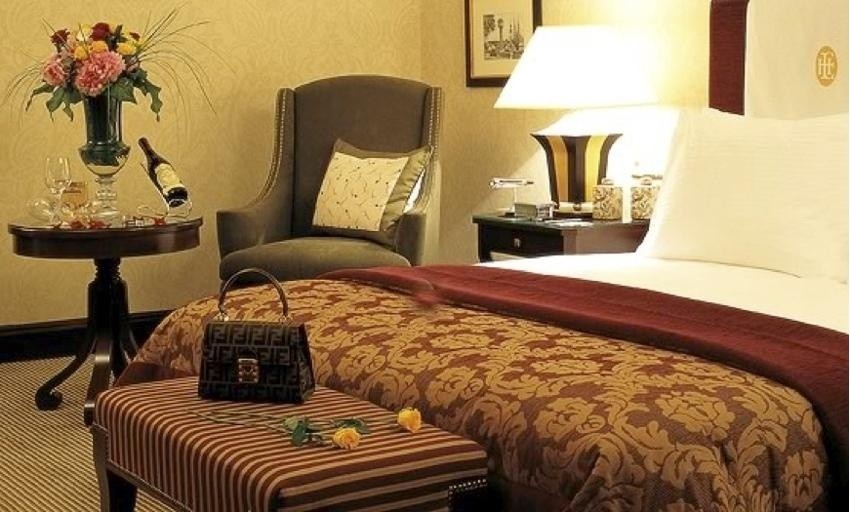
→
[195,265,322,407]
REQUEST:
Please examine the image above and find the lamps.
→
[492,25,660,222]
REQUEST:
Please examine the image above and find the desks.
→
[8,215,202,426]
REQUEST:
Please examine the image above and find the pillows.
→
[651,107,848,281]
[312,137,438,246]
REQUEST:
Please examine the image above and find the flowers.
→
[4,9,235,168]
[188,407,421,451]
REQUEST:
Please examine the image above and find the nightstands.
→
[472,212,650,263]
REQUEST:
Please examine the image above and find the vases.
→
[77,93,131,215]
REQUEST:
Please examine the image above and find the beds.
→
[114,252,849,512]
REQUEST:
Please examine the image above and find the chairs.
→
[217,74,446,296]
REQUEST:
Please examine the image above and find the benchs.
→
[92,375,488,512]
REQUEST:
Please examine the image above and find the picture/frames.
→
[464,0,541,87]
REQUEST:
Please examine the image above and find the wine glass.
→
[44,155,71,226]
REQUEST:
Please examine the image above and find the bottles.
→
[630,176,662,220]
[590,177,625,219]
[137,136,188,208]
